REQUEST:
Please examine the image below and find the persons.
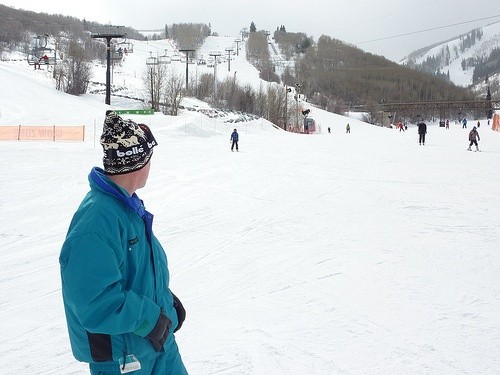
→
[446,120,449,129]
[346,123,350,133]
[462,119,467,128]
[477,120,480,127]
[58,109,189,375]
[328,127,330,133]
[468,126,480,151]
[39,55,49,72]
[231,129,239,152]
[398,122,405,132]
[118,47,127,56]
[418,120,427,146]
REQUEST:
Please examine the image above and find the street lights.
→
[91,32,125,104]
[234,41,242,56]
[178,49,195,90]
[210,54,221,93]
[225,49,234,72]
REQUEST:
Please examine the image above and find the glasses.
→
[139,123,158,146]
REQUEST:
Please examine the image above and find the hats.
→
[101,109,152,173]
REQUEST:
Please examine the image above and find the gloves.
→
[146,315,172,351]
[172,291,185,333]
